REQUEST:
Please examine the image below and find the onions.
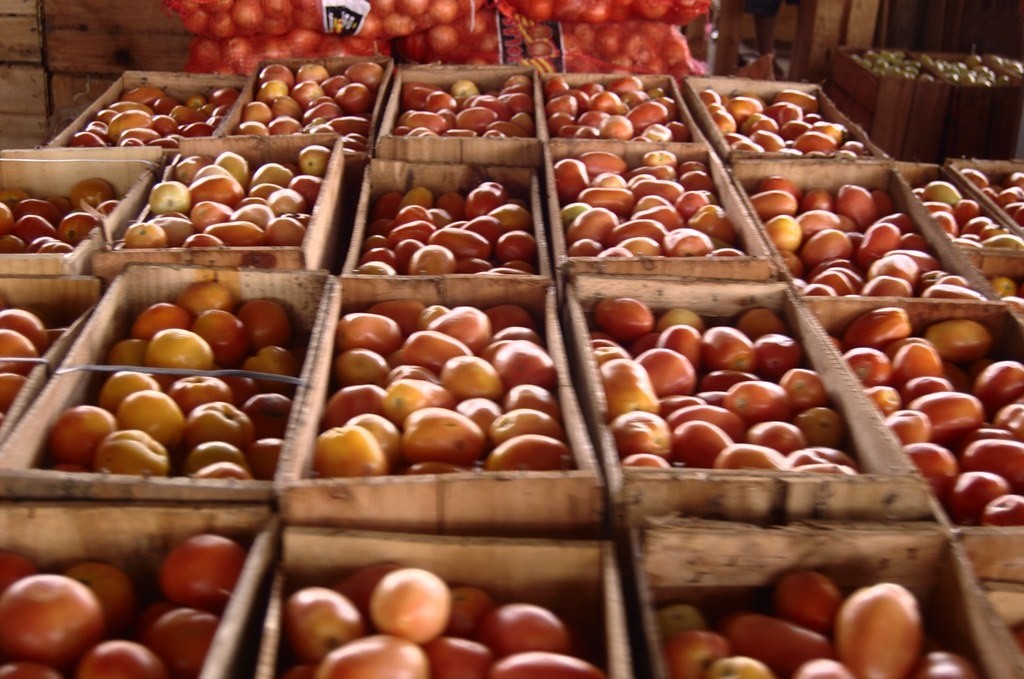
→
[171,0,690,75]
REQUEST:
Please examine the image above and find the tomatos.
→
[0,60,1024,679]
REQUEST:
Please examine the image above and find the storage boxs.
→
[0,0,1024,679]
